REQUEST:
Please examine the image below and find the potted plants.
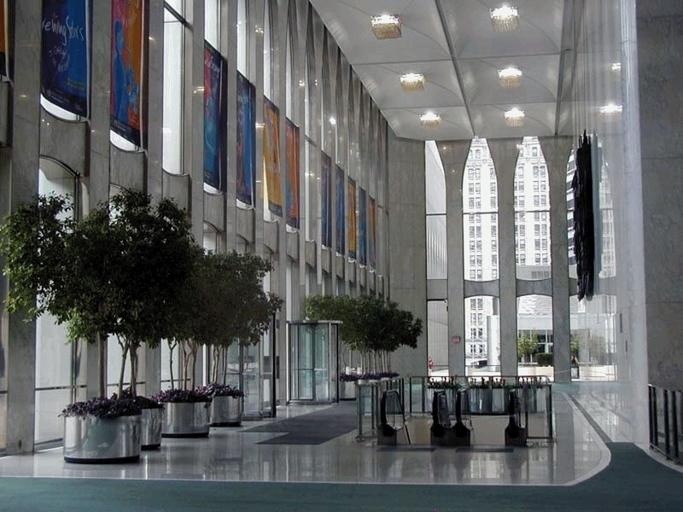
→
[304,292,423,401]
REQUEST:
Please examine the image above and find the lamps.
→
[369,1,525,128]
